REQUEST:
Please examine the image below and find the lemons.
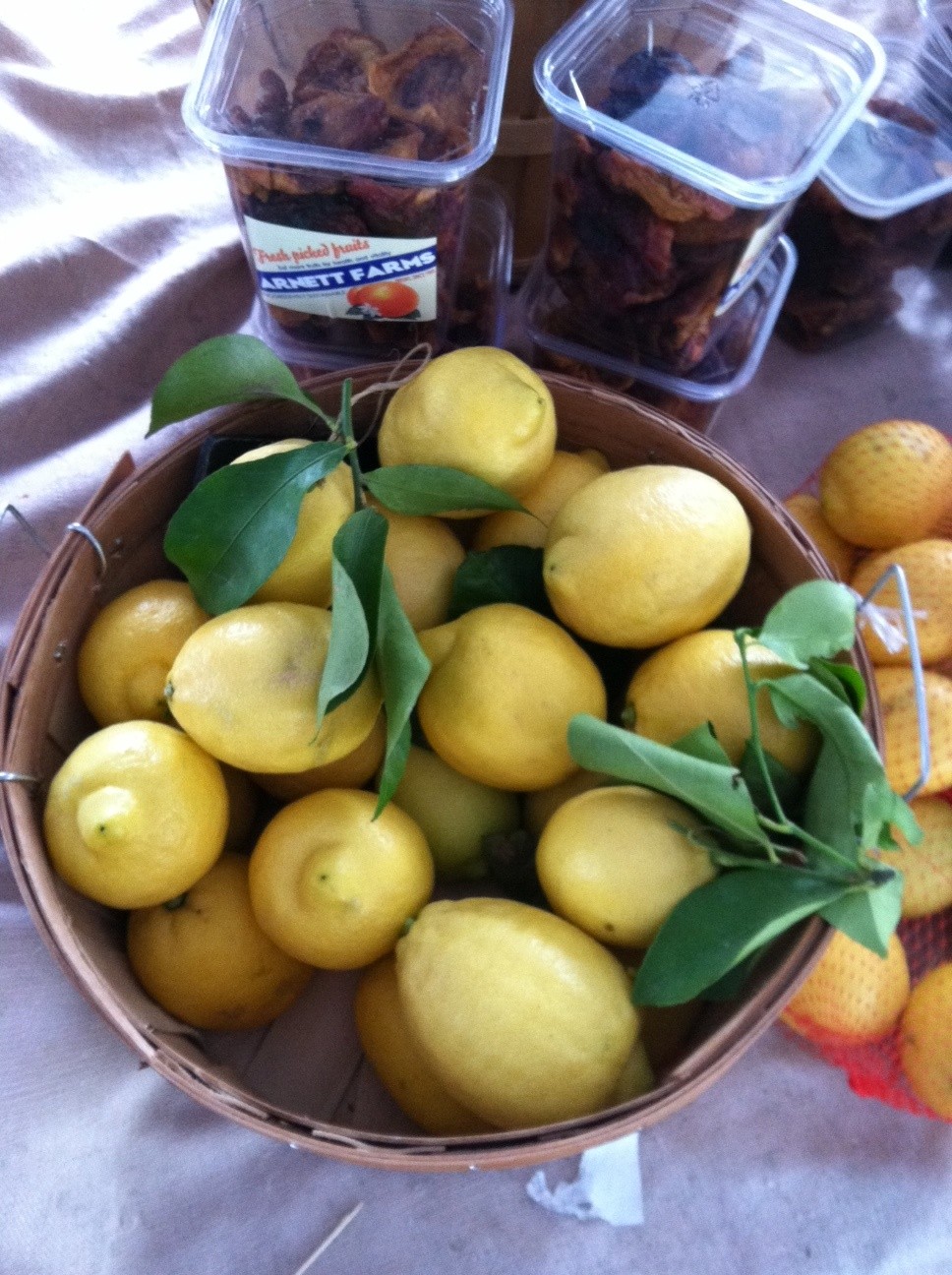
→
[772,418,951,1121]
[41,342,815,1136]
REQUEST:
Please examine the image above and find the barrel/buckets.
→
[0,355,931,1171]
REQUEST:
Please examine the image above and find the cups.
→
[178,0,950,439]
